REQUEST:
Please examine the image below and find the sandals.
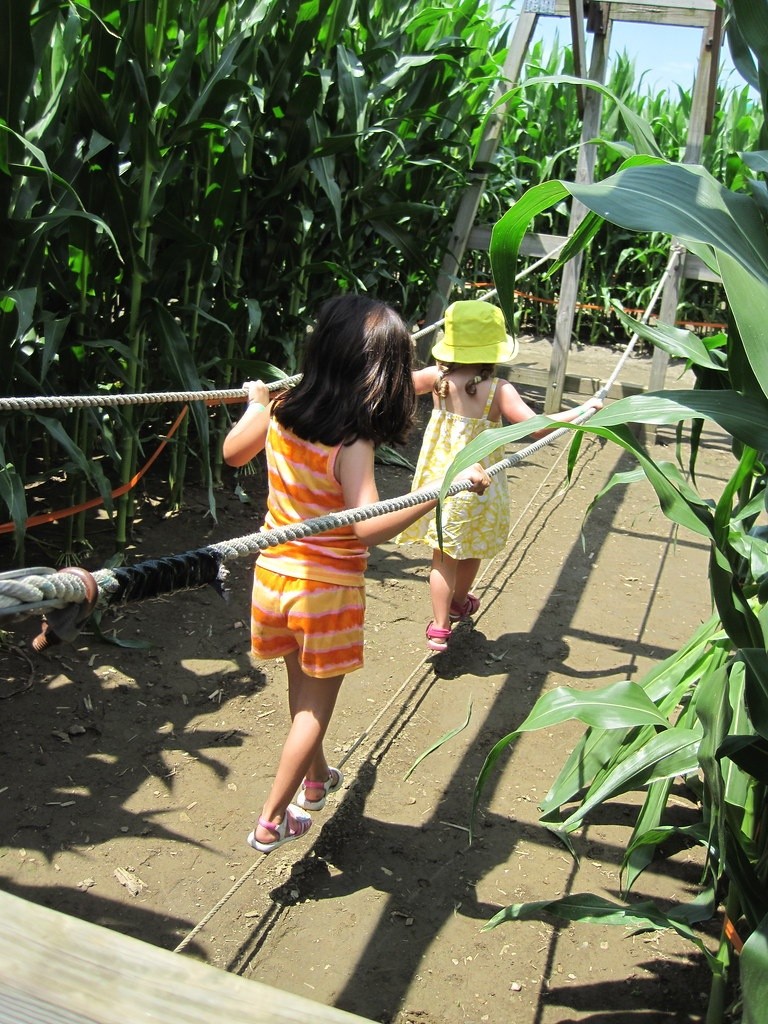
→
[248,803,313,853]
[449,595,480,622]
[298,766,344,811]
[425,619,452,651]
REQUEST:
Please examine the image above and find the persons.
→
[223,296,492,850]
[412,299,603,650]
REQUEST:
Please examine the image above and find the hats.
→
[432,300,519,364]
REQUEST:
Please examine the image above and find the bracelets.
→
[577,406,586,414]
[249,404,264,412]
